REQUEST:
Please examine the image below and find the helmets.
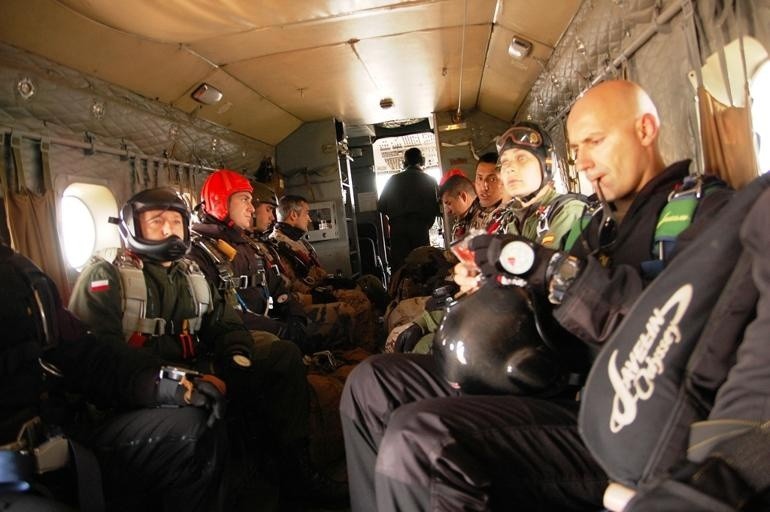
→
[200,170,279,227]
[108,187,190,261]
[434,285,563,398]
[495,122,558,185]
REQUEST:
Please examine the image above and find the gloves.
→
[468,234,556,289]
[394,324,422,354]
[312,277,356,303]
[159,352,253,427]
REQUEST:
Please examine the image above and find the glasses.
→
[497,127,542,151]
[580,178,619,269]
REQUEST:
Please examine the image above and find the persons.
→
[379,146,514,358]
[65,185,350,509]
[2,234,239,509]
[379,117,599,353]
[187,167,389,483]
[574,168,770,509]
[337,77,734,511]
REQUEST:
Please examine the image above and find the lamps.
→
[507,35,532,61]
[191,82,224,106]
